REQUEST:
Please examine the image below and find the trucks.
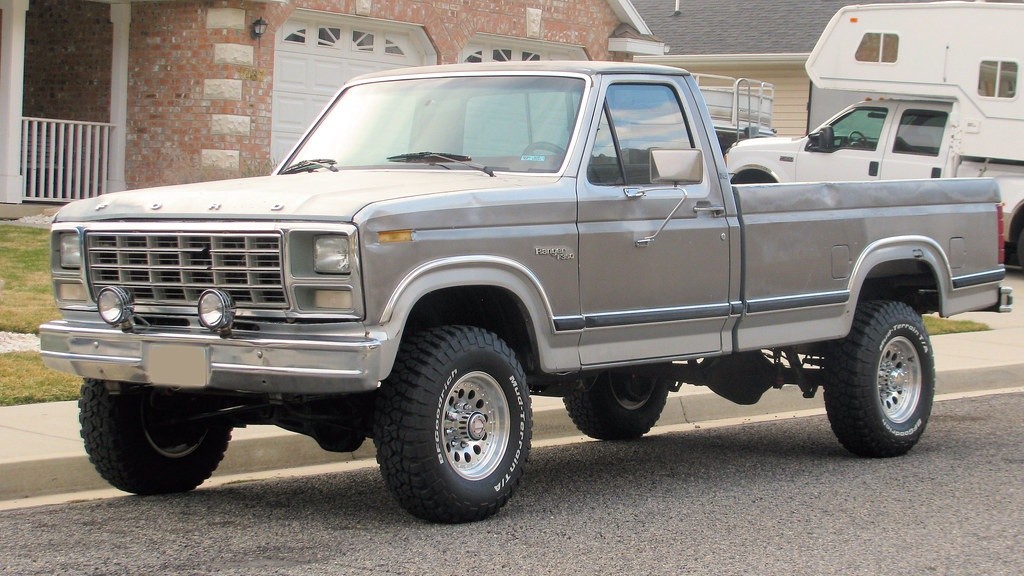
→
[724,0,1024,271]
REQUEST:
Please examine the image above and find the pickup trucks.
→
[39,61,1013,523]
